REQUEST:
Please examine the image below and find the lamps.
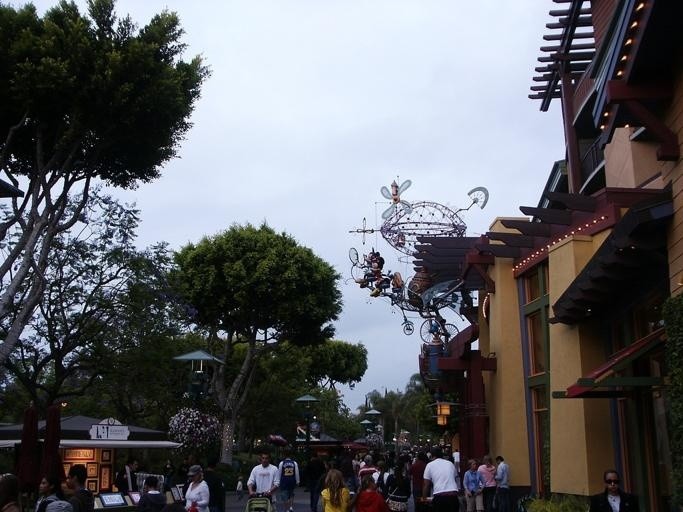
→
[425,396,463,417]
[429,414,451,427]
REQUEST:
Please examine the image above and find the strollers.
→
[244,493,274,512]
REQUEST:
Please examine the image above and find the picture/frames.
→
[170,484,186,503]
[62,446,114,494]
[98,490,141,506]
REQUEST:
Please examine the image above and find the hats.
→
[186,465,202,476]
[358,467,376,477]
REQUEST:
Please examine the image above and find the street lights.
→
[296,393,321,489]
[173,349,225,412]
[361,408,432,455]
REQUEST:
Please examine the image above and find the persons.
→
[1,432,509,509]
[589,470,638,512]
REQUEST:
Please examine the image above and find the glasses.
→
[605,479,619,485]
